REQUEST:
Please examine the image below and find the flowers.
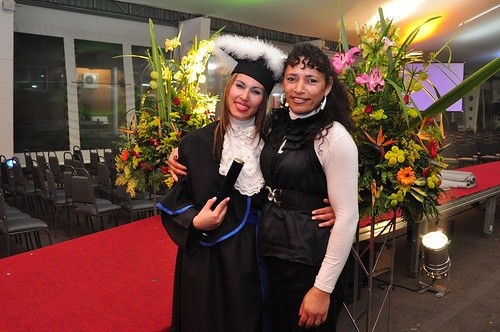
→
[111,18,227,199]
[331,0,500,227]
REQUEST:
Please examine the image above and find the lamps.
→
[419,228,451,278]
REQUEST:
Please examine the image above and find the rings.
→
[315,320,322,326]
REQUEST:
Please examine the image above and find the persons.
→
[168,43,360,332]
[156,34,337,332]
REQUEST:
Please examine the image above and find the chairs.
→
[0,146,166,257]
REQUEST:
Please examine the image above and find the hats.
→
[210,34,287,96]
